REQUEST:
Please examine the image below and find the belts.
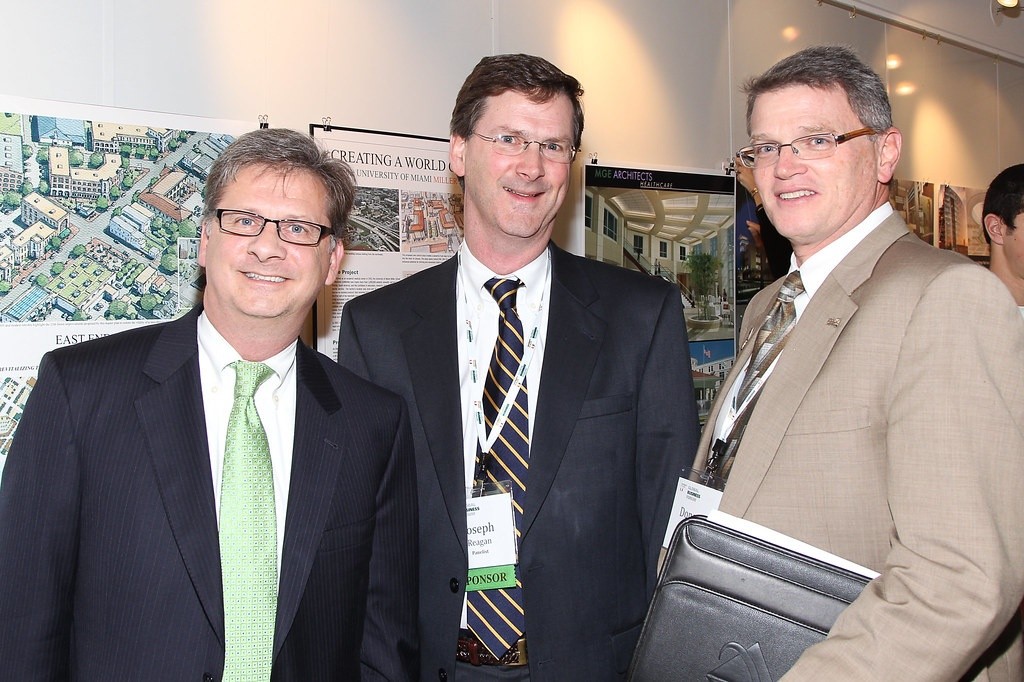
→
[456,636,527,667]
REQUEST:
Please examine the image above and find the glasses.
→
[471,130,582,164]
[735,126,877,169]
[213,208,336,247]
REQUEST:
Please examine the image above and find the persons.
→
[0,130,420,681]
[337,55,701,681]
[657,44,1024,682]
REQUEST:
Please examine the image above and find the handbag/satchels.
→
[626,513,875,682]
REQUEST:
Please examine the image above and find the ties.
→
[707,270,805,492]
[222,359,278,681]
[466,276,530,661]
